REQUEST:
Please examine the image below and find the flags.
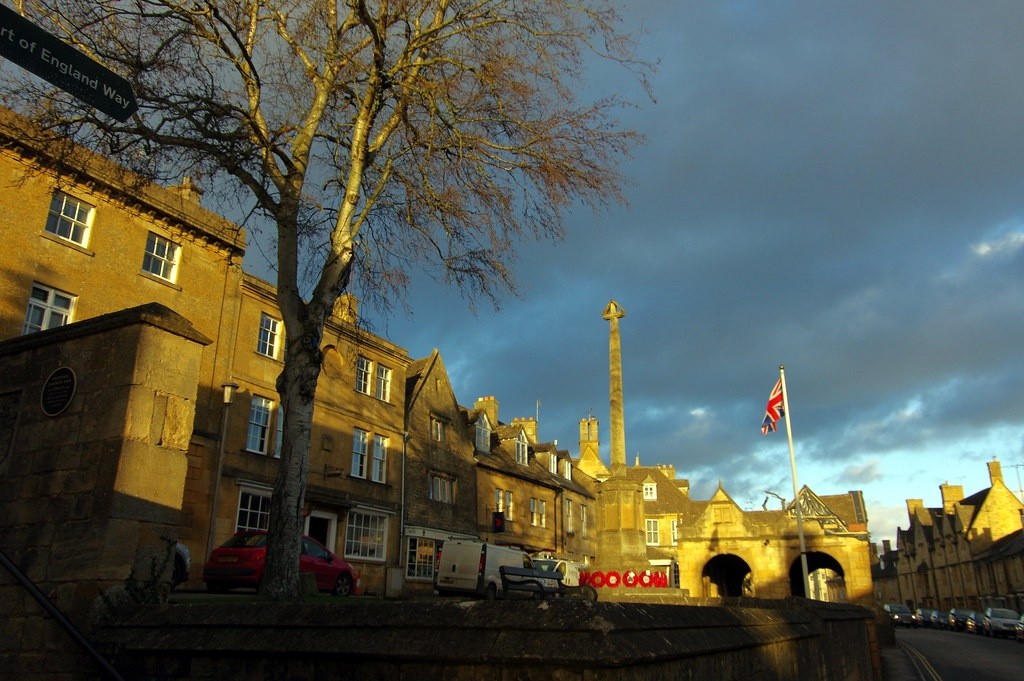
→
[760,378,786,435]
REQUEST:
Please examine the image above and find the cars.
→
[949,608,968,631]
[884,603,917,628]
[203,529,357,596]
[983,608,1018,639]
[916,608,930,627]
[966,609,985,635]
[1015,614,1024,641]
[930,610,948,629]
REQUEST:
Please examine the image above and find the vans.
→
[432,539,536,600]
